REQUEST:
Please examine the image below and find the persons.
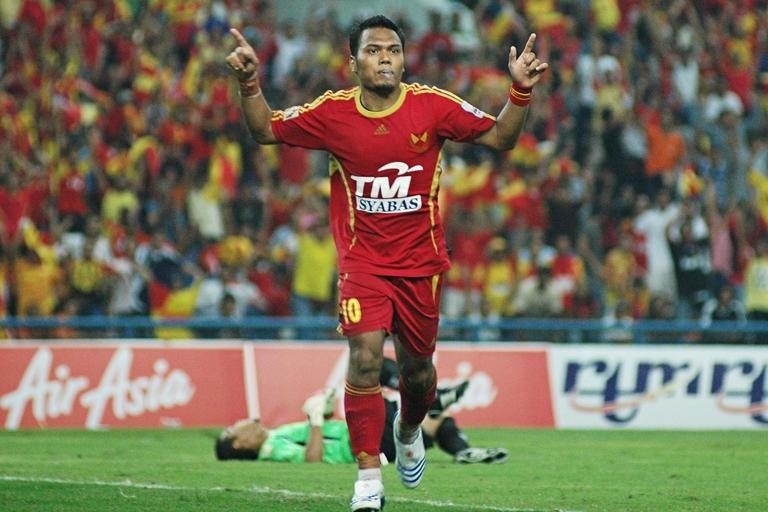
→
[215,354,507,462]
[226,15,549,512]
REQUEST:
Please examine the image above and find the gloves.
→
[302,388,336,427]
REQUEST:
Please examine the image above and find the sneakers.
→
[454,447,512,465]
[428,380,470,419]
[350,479,385,512]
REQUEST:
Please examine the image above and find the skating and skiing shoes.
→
[391,410,426,490]
